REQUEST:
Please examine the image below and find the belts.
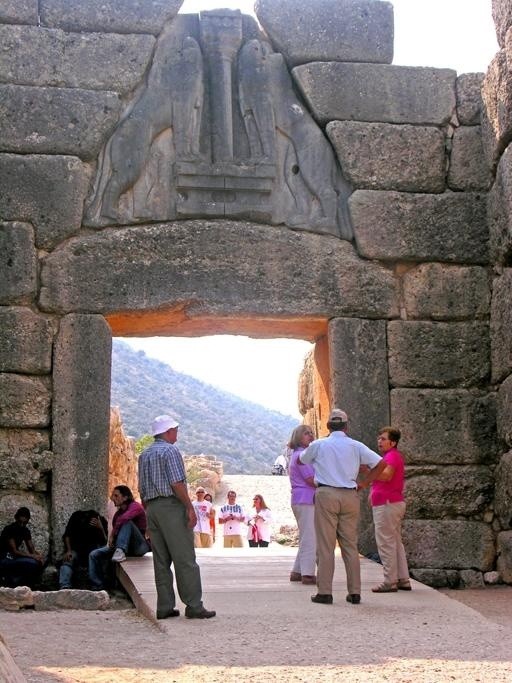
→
[317,482,356,489]
[146,495,174,503]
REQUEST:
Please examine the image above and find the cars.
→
[271,453,289,475]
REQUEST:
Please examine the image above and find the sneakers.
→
[111,548,127,563]
[59,585,70,589]
[86,584,105,591]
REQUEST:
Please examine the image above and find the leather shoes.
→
[156,608,180,620]
[346,594,362,603]
[311,594,334,604]
[184,605,217,619]
[290,571,302,581]
[301,575,317,584]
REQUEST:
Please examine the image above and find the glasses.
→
[197,492,204,493]
[304,431,312,435]
[377,436,391,441]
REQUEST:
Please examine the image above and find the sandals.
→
[397,580,411,591]
[372,581,398,592]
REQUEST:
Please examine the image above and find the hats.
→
[328,409,348,423]
[151,414,180,437]
